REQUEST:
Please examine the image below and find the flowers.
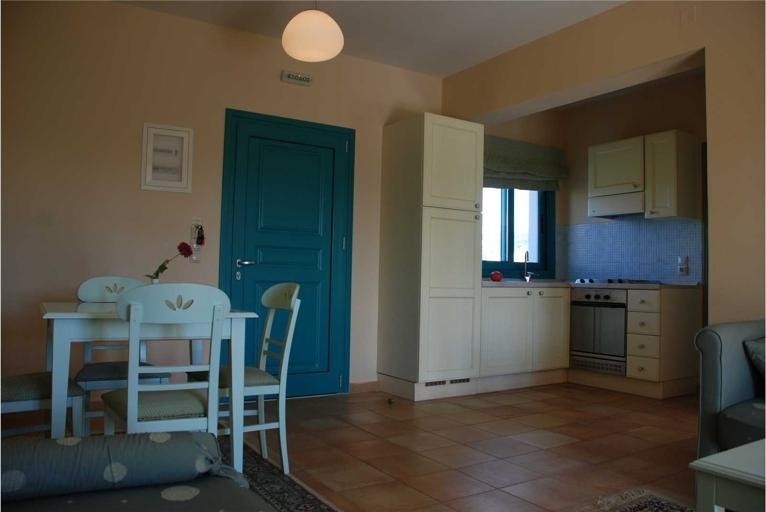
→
[144,241,193,278]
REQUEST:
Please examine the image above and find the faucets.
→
[525,251,528,274]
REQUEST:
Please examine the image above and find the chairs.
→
[101,281,231,440]
[695,320,766,457]
[72,275,171,436]
[185,281,301,476]
[1,372,86,437]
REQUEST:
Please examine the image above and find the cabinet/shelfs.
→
[381,113,481,384]
[643,130,701,219]
[626,287,697,380]
[482,280,570,377]
[585,136,644,216]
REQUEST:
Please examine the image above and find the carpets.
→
[564,484,699,511]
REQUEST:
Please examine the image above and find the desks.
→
[40,300,258,475]
[691,437,765,512]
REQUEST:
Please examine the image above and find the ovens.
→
[570,289,626,358]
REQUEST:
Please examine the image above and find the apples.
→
[491,271,502,281]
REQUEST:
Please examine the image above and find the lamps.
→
[280,1,346,63]
[192,222,205,264]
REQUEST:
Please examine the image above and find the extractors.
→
[587,192,644,219]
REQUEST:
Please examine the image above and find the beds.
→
[1,430,275,511]
